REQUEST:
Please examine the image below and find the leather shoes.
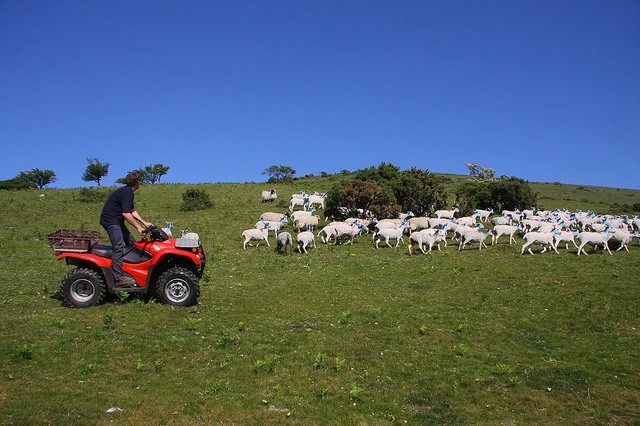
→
[116,279,135,288]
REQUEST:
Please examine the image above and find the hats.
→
[123,173,140,184]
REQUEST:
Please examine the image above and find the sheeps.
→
[308,193,328,210]
[451,223,484,242]
[292,190,309,199]
[491,224,521,245]
[261,189,278,203]
[491,213,514,226]
[254,218,288,238]
[503,212,525,224]
[313,191,319,195]
[434,207,460,220]
[554,230,580,250]
[427,217,457,229]
[290,208,317,222]
[408,230,441,256]
[317,206,378,247]
[374,218,407,230]
[396,211,415,222]
[288,197,308,212]
[456,213,481,227]
[457,229,493,252]
[521,228,561,255]
[408,217,429,234]
[502,208,521,216]
[296,230,317,254]
[292,215,321,232]
[420,224,451,248]
[259,212,290,221]
[577,232,613,256]
[521,207,639,235]
[474,207,494,223]
[276,230,293,256]
[241,222,271,250]
[609,229,631,252]
[374,221,412,249]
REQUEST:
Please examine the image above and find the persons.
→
[99,172,155,288]
